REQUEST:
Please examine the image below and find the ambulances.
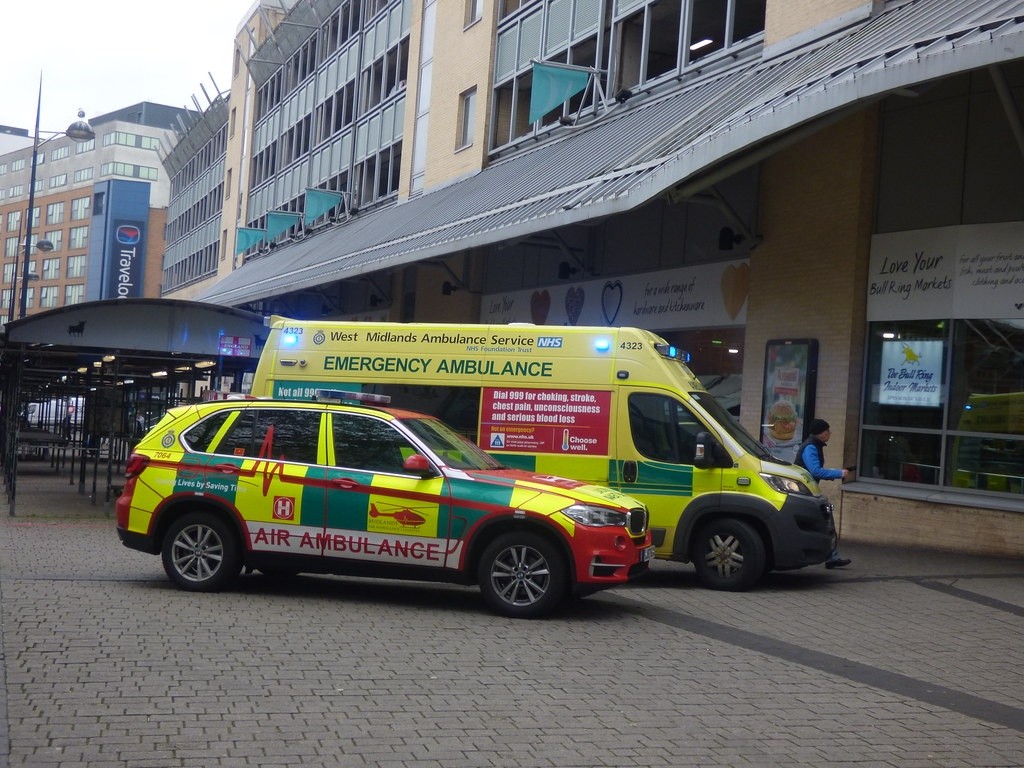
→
[116,389,656,615]
[948,392,1024,498]
[248,314,835,594]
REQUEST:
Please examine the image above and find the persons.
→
[136,410,145,432]
[793,418,852,569]
[62,413,73,440]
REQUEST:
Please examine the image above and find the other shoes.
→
[825,557,852,569]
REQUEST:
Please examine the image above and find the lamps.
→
[558,260,580,279]
[718,226,746,250]
[321,304,334,315]
[442,281,460,295]
[614,89,633,104]
[369,294,382,307]
[350,207,359,216]
[558,116,575,126]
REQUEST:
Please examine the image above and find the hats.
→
[810,418,830,435]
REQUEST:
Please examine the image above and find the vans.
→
[20,397,84,434]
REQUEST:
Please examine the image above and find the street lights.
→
[20,119,98,316]
[9,274,40,320]
[8,241,54,321]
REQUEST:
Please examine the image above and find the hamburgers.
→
[769,400,797,440]
